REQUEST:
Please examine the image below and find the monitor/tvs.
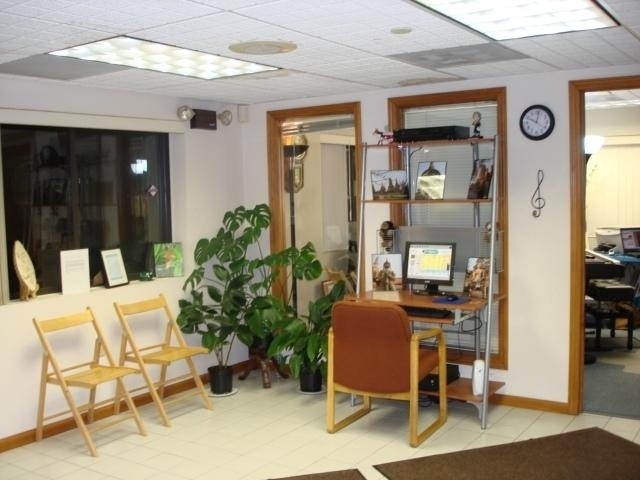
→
[403,241,456,295]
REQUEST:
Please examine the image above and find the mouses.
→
[447,295,458,301]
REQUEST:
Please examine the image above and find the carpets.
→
[372,427,640,479]
[264,468,367,480]
[584,334,640,351]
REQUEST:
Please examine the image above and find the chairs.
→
[325,301,447,448]
[114,293,214,428]
[32,307,143,456]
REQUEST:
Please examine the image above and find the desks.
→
[339,286,508,431]
[585,251,638,337]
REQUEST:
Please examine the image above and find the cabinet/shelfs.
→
[360,135,500,207]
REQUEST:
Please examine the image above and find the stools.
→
[585,279,637,352]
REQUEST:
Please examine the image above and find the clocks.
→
[519,105,555,141]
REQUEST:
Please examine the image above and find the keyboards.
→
[401,307,451,318]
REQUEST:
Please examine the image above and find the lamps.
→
[584,135,606,164]
[283,143,309,320]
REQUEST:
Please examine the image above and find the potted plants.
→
[178,205,322,394]
[176,287,252,395]
[269,281,346,393]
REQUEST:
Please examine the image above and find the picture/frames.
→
[98,246,130,289]
